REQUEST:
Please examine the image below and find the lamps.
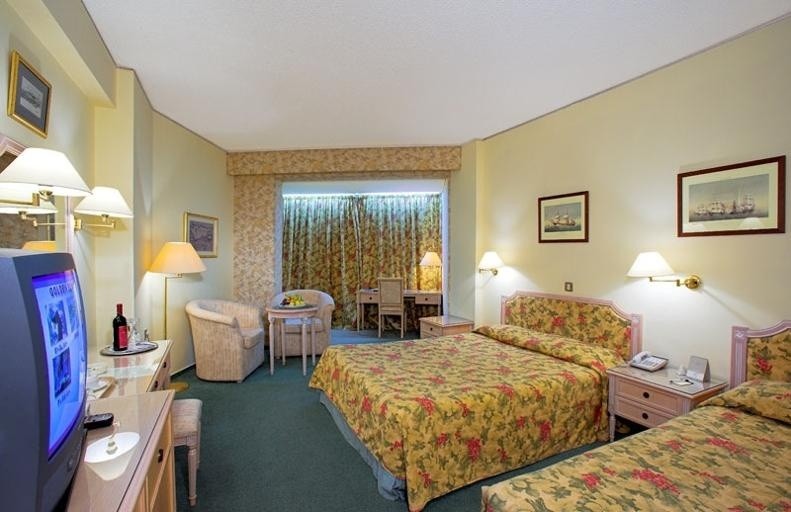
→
[22,240,58,252]
[419,251,442,266]
[0,148,95,210]
[76,186,135,231]
[148,241,207,393]
[1,198,67,228]
[626,252,701,290]
[479,251,502,275]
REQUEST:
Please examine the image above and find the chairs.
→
[271,289,336,361]
[378,278,407,338]
[185,299,266,384]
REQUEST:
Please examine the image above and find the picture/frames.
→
[184,212,219,258]
[537,190,589,243]
[677,153,787,238]
[8,50,54,138]
[1,135,76,255]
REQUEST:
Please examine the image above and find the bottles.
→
[112,303,128,351]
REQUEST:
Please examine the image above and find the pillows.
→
[701,378,791,426]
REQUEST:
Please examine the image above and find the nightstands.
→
[418,314,474,338]
[606,363,730,444]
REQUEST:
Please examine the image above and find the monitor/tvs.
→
[0,247,88,512]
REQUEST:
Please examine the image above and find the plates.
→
[279,303,306,308]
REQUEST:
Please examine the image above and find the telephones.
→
[628,351,669,373]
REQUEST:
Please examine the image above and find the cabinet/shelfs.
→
[86,339,176,400]
[65,389,179,512]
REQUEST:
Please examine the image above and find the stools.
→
[171,399,207,507]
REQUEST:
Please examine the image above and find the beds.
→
[481,319,791,511]
[309,291,644,500]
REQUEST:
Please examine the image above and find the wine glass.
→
[127,319,141,347]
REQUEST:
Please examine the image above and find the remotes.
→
[83,412,114,428]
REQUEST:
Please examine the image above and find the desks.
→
[266,306,321,377]
[355,288,442,333]
